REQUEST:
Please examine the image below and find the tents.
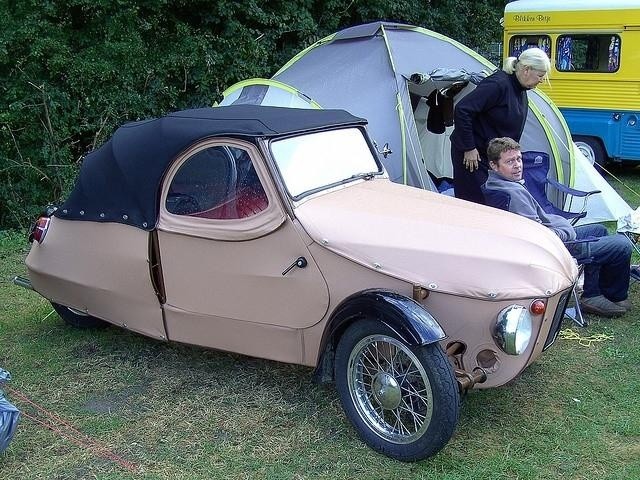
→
[212,20,633,228]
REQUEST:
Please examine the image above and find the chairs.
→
[480,150,602,327]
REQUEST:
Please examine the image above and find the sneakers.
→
[579,295,633,318]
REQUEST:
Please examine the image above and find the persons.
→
[448,48,551,205]
[486,135,634,316]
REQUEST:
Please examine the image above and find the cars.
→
[12,105,578,463]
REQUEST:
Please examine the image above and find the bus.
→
[502,1,640,176]
